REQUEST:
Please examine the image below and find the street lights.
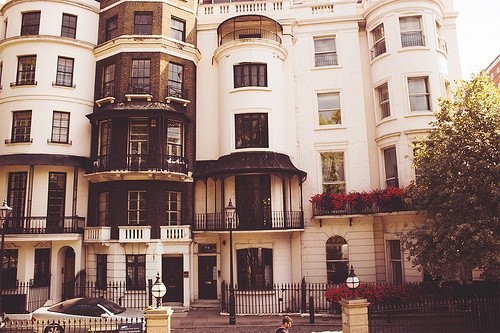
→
[0,198,14,323]
[222,198,238,326]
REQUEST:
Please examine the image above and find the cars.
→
[33,295,147,333]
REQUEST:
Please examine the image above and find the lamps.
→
[345,264,359,289]
[150,273,167,298]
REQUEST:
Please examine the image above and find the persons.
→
[276,315,293,333]
